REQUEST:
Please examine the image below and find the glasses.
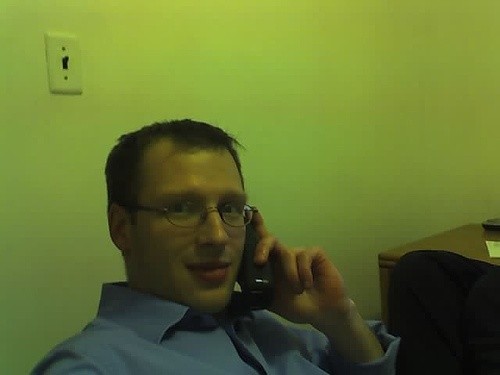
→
[129,200,257,228]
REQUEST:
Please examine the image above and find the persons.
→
[32,119,402,375]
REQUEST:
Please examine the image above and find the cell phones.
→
[237,215,273,310]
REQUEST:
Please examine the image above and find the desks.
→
[376,222,500,331]
